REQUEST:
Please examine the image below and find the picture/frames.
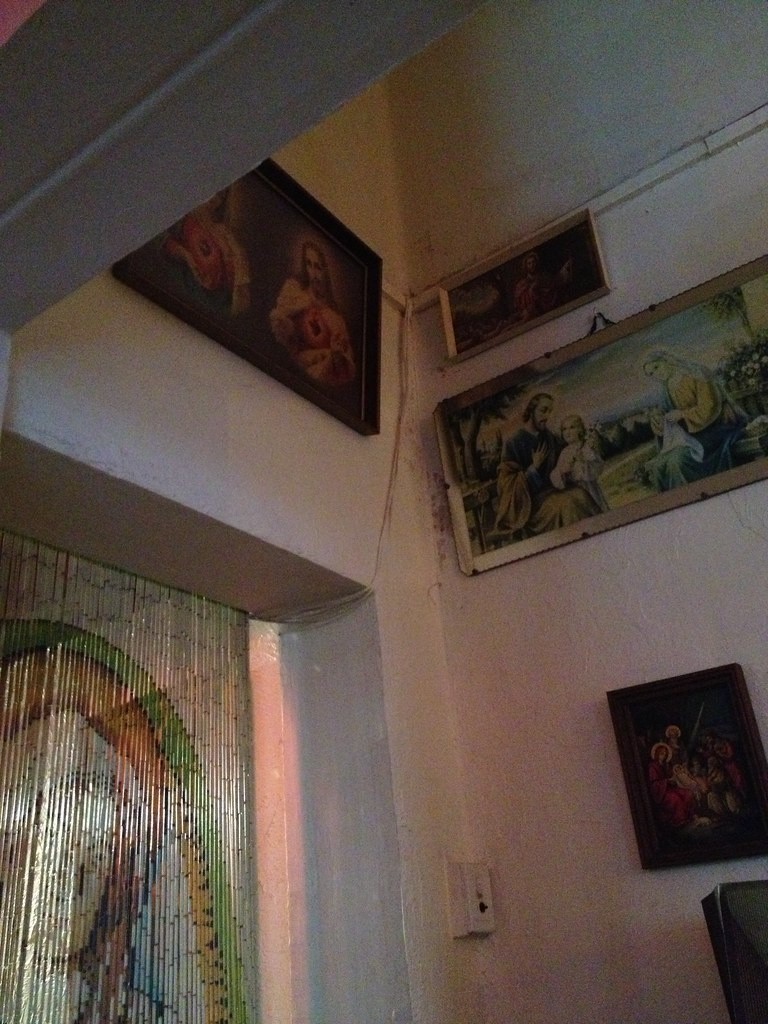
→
[430,250,768,577]
[112,156,384,436]
[436,208,612,364]
[606,662,768,870]
